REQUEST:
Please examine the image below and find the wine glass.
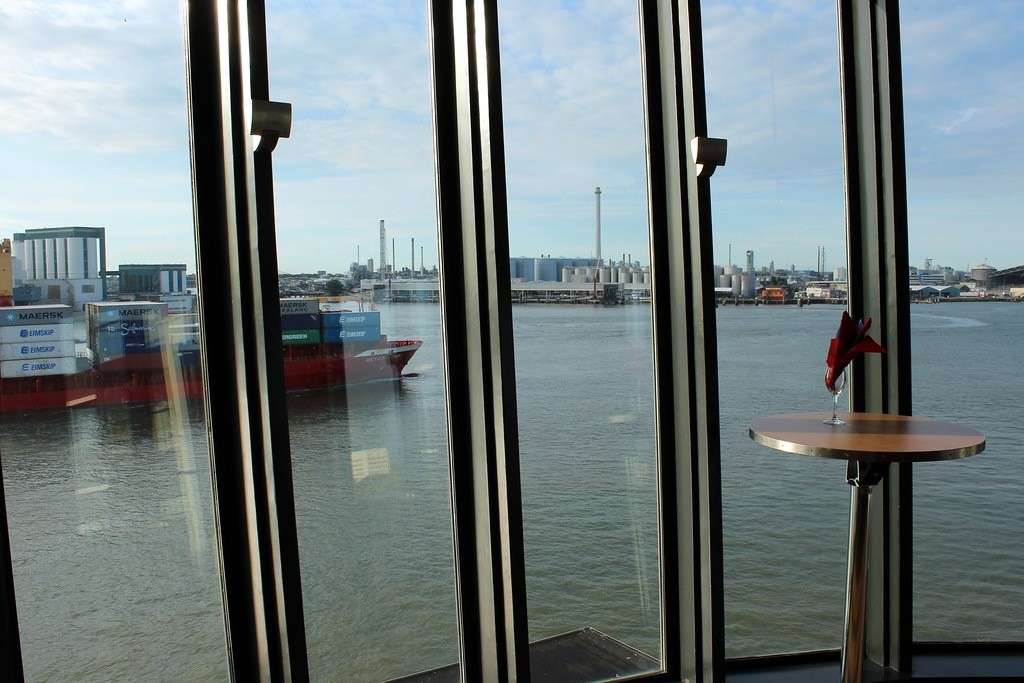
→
[822,367,847,425]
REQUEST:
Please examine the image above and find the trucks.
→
[761,288,783,300]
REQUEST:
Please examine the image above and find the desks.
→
[748,413,987,683]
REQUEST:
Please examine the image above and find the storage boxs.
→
[279,297,380,345]
[0,300,201,377]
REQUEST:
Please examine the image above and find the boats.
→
[589,296,600,304]
[0,264,423,414]
